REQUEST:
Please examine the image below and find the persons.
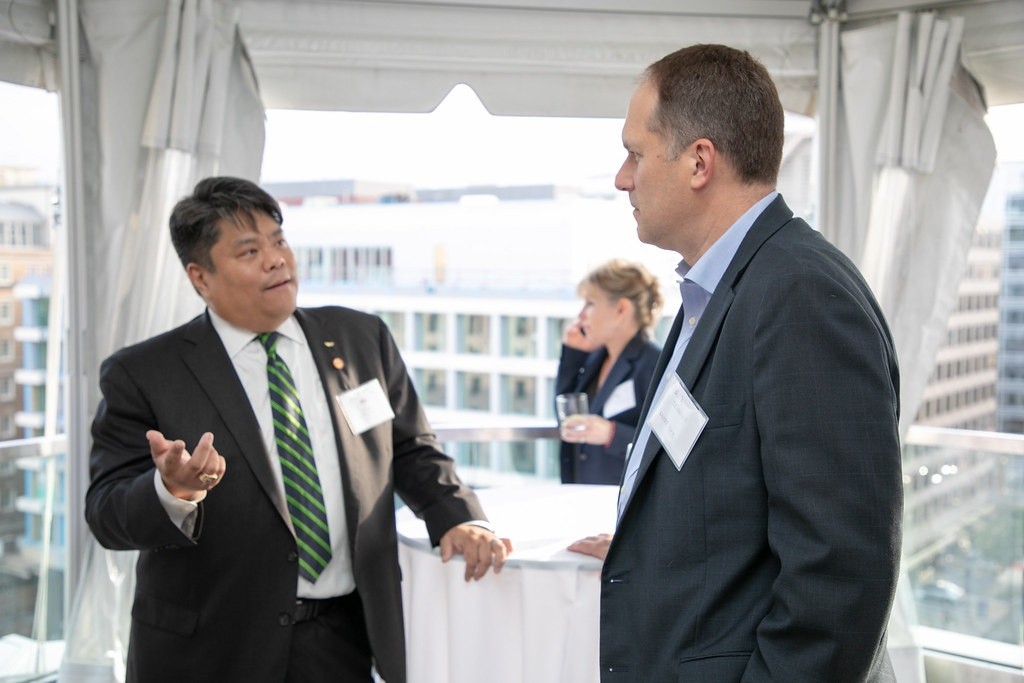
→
[83,176,513,683]
[567,45,904,683]
[554,260,664,486]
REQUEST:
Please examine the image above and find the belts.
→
[293,594,358,623]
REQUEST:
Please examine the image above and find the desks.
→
[394,485,925,681]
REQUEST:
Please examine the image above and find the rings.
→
[198,471,218,484]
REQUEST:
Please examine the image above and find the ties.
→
[257,331,333,584]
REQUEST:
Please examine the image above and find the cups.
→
[556,392,590,423]
